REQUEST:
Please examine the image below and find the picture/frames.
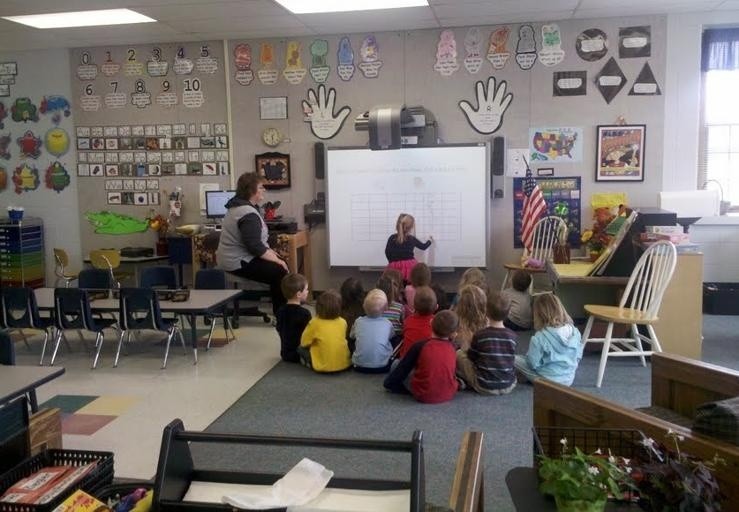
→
[75,122,231,207]
[258,96,290,120]
[0,62,18,98]
[594,123,647,182]
[254,152,292,191]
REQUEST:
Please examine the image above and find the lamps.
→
[659,188,721,232]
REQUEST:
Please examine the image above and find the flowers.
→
[537,426,724,511]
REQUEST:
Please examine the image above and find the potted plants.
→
[262,200,281,219]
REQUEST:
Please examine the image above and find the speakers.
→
[313,141,326,209]
[490,135,506,200]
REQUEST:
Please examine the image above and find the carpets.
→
[141,293,738,512]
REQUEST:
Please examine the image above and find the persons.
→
[381,310,465,403]
[499,270,532,332]
[213,170,288,327]
[274,270,310,359]
[301,262,488,376]
[454,289,517,395]
[512,293,585,389]
[385,212,433,282]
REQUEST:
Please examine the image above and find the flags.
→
[519,166,546,270]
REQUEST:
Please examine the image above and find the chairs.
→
[52,247,80,287]
[89,248,128,298]
[521,348,738,512]
[3,265,270,370]
[498,215,570,299]
[575,239,678,387]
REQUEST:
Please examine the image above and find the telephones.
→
[303,192,326,225]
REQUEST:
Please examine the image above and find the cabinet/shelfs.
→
[0,216,45,293]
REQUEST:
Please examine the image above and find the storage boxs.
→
[701,281,739,315]
[624,204,677,234]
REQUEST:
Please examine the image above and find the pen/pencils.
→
[169,192,185,201]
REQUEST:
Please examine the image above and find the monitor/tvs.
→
[205,190,236,224]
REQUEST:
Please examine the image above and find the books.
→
[583,248,612,278]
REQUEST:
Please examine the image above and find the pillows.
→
[691,394,739,448]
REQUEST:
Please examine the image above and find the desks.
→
[503,465,557,512]
[194,231,314,302]
[83,254,168,287]
[1,364,66,489]
[546,260,652,364]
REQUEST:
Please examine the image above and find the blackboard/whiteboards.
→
[323,142,491,273]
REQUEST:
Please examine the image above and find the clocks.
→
[262,126,283,147]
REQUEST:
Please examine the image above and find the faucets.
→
[702,178,739,217]
